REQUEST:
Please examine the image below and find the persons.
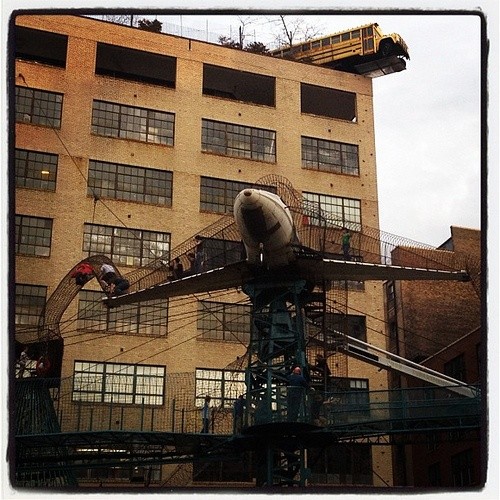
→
[200,395,211,432]
[35,354,51,377]
[316,355,331,375]
[340,226,352,260]
[18,346,29,379]
[308,386,321,425]
[171,235,205,280]
[285,367,307,423]
[233,394,246,435]
[70,262,130,298]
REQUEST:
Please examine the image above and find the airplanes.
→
[101,189,472,309]
